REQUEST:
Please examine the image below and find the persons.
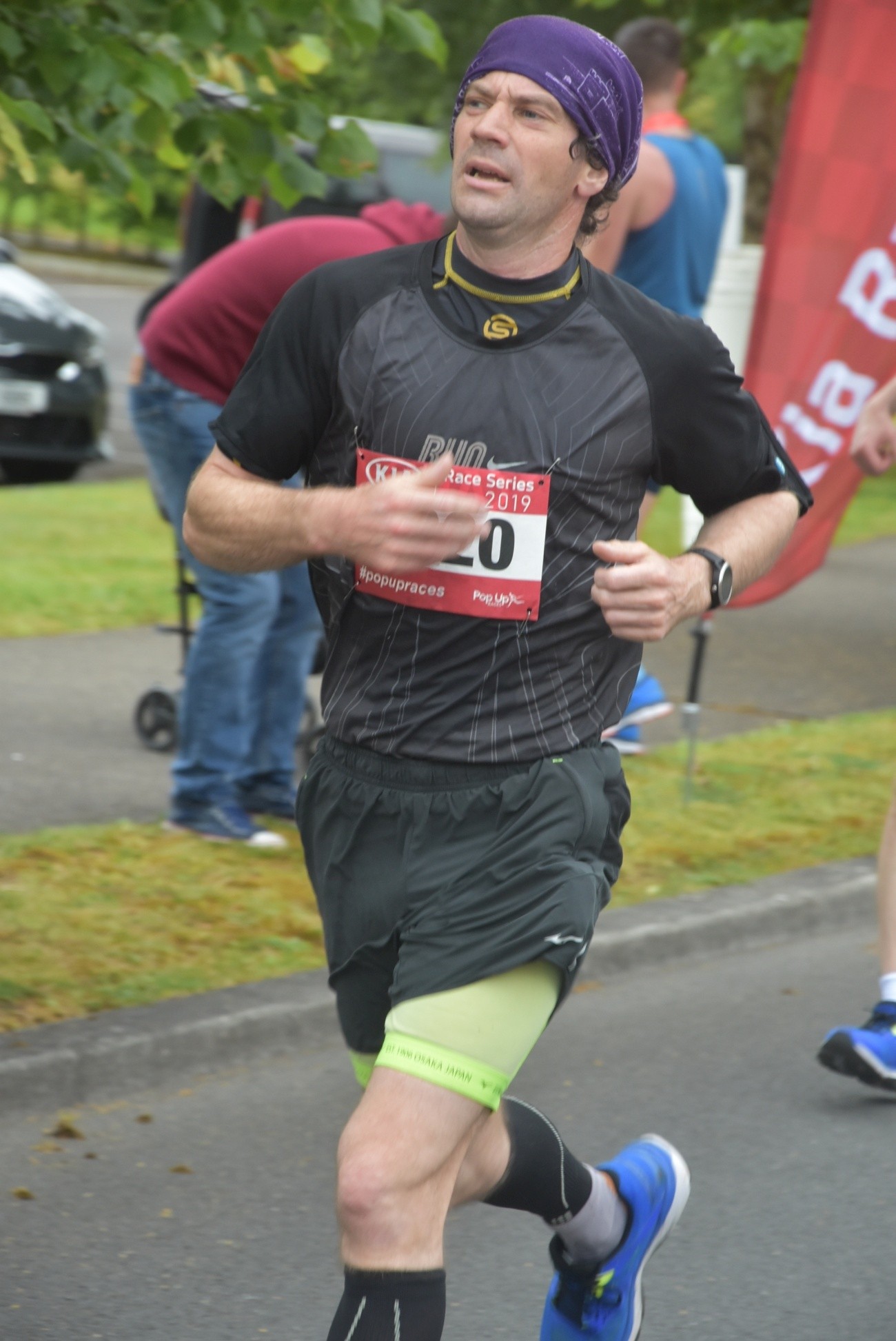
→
[583,15,729,756]
[182,13,813,1341]
[815,375,895,1092]
[128,199,444,852]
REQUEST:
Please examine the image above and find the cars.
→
[0,251,108,482]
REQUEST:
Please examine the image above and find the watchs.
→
[683,548,734,612]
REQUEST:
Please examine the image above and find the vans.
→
[132,73,457,342]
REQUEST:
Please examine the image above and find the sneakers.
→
[609,726,643,755]
[817,1001,896,1087]
[602,667,669,743]
[161,807,285,851]
[540,1134,690,1341]
